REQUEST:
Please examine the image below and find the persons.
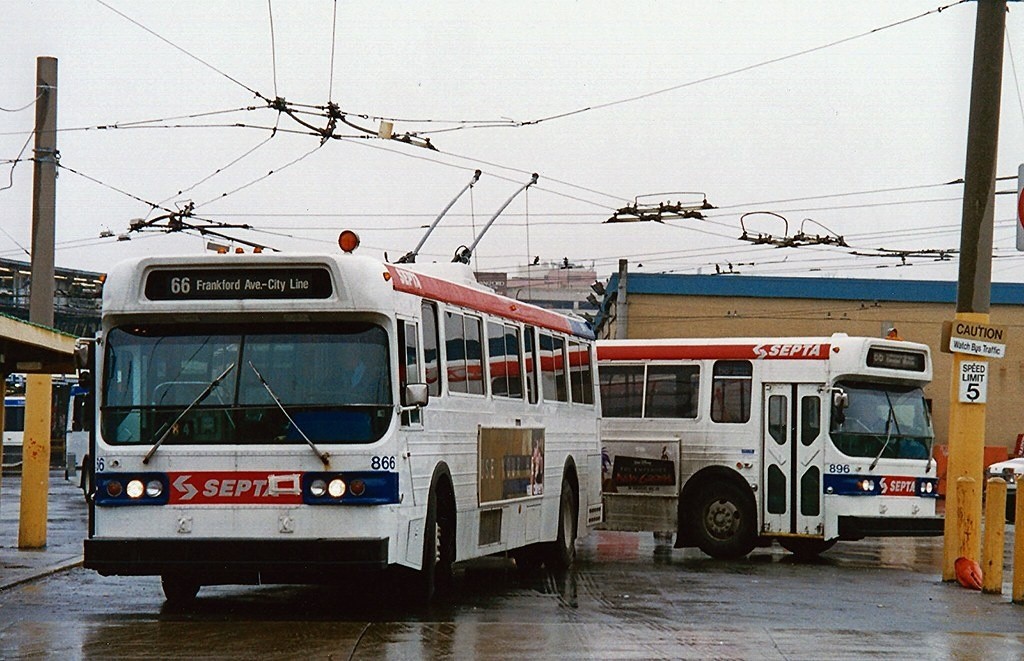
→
[531,440,545,495]
[322,335,377,396]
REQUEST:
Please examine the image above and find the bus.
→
[596,327,946,560]
[2,376,92,502]
[71,230,606,612]
